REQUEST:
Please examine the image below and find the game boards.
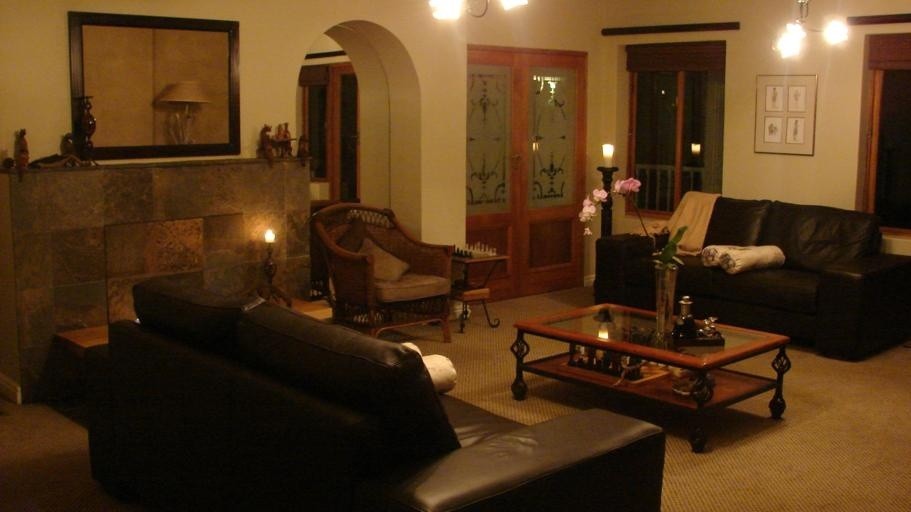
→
[451,256,510,264]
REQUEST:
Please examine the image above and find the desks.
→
[56,293,334,415]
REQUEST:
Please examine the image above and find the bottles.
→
[703,317,716,337]
[675,296,693,337]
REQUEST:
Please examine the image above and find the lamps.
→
[428,0,528,21]
[155,80,212,145]
[774,0,849,58]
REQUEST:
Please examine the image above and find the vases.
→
[654,263,677,334]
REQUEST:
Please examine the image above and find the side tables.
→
[454,256,510,334]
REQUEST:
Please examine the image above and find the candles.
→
[603,143,614,168]
[264,229,275,244]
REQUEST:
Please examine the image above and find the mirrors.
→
[67,10,240,160]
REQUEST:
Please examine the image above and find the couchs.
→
[593,190,911,361]
[88,271,665,512]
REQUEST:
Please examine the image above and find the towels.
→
[666,191,722,256]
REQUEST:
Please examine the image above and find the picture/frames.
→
[752,73,818,156]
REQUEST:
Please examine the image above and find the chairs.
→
[312,203,455,344]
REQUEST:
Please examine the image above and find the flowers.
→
[578,176,689,270]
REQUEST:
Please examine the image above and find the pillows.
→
[719,245,786,274]
[400,342,423,356]
[357,237,409,281]
[420,354,456,393]
[701,244,757,267]
